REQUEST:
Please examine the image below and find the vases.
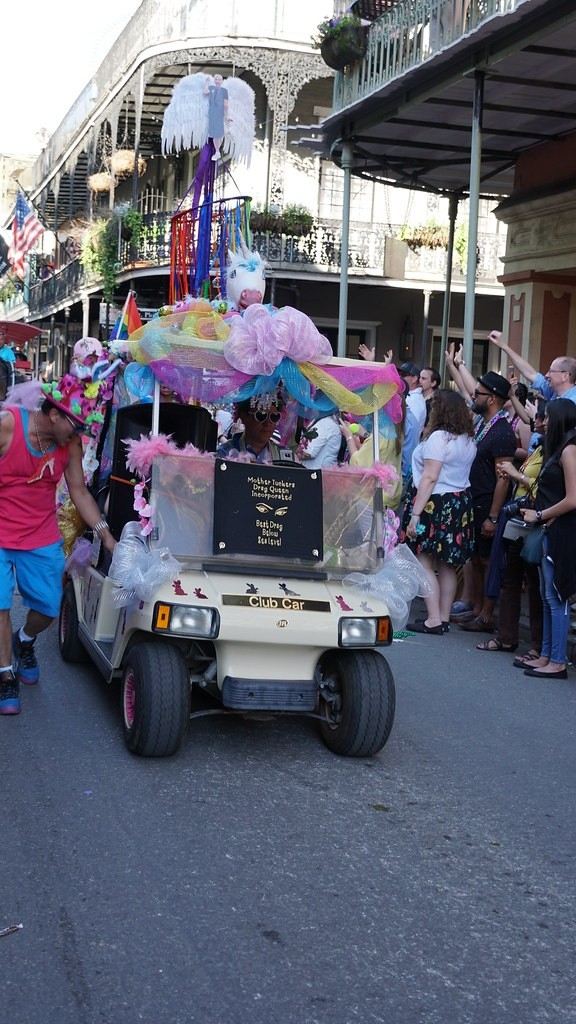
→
[385,236,462,280]
[121,222,133,242]
[271,225,312,237]
[320,27,366,70]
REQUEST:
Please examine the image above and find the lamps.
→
[399,315,415,362]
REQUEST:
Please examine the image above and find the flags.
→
[7,189,46,279]
[0,235,13,279]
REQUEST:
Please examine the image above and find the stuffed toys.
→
[224,227,272,313]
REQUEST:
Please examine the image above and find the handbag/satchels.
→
[519,525,547,563]
[502,517,532,563]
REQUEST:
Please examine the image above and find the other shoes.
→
[523,668,568,679]
[460,617,495,633]
[406,622,444,635]
[415,619,449,631]
[513,660,538,669]
[449,600,473,616]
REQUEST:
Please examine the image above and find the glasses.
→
[548,368,571,376]
[248,410,281,424]
[62,414,86,435]
[475,390,492,397]
[399,373,414,378]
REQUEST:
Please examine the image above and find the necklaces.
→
[474,409,504,444]
[239,432,272,461]
[27,410,55,483]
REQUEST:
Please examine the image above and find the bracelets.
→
[218,434,223,441]
[456,360,465,368]
[94,520,109,532]
[521,475,525,484]
[411,514,420,516]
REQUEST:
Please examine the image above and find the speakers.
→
[95,402,219,579]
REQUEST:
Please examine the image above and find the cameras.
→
[504,495,533,518]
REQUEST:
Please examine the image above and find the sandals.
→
[515,652,539,661]
[476,639,519,651]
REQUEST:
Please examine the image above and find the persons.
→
[0,374,118,715]
[46,262,54,273]
[132,360,188,405]
[215,380,295,466]
[0,332,16,363]
[204,74,233,161]
[296,330,576,679]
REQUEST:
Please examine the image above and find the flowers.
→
[401,221,469,275]
[295,428,319,461]
[385,508,400,557]
[309,8,362,48]
[250,201,313,233]
[56,340,129,509]
[133,476,155,536]
[98,201,143,304]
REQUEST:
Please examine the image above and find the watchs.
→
[536,511,542,521]
[488,516,498,524]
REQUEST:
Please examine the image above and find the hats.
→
[41,374,97,425]
[477,371,512,400]
[397,362,421,376]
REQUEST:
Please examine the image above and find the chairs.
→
[105,400,216,572]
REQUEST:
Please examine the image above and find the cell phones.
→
[509,366,515,378]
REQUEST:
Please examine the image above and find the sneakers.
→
[11,627,40,685]
[0,669,20,714]
